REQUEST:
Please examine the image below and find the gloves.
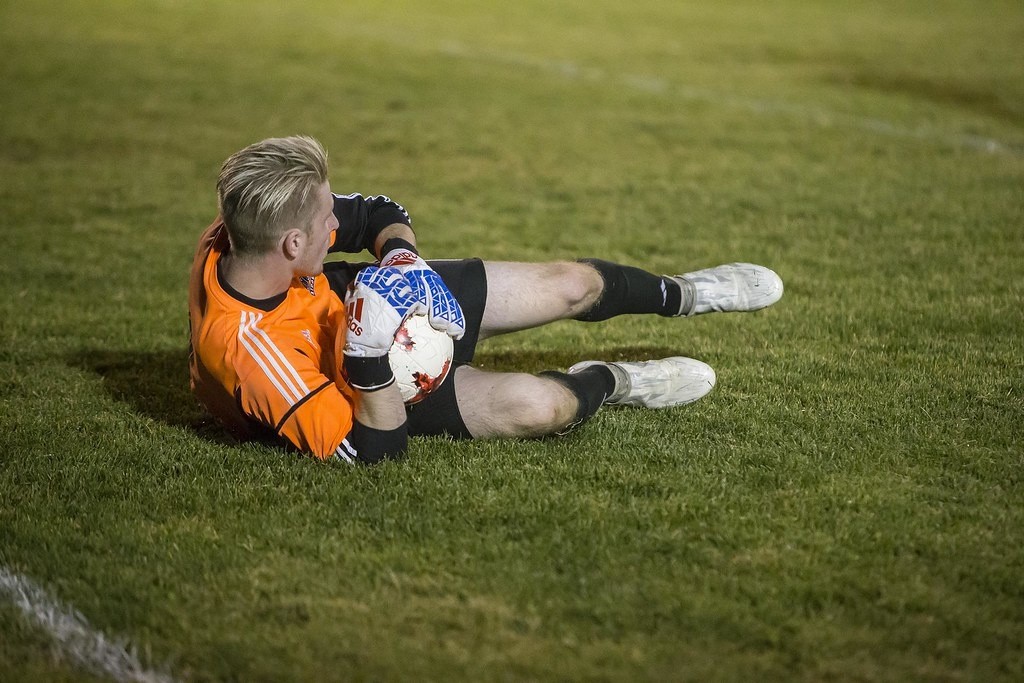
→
[342,267,420,392]
[379,238,466,341]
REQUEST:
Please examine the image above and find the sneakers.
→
[567,357,716,409]
[674,262,783,318]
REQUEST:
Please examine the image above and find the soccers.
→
[334,314,454,407]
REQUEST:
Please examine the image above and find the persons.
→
[187,134,785,465]
